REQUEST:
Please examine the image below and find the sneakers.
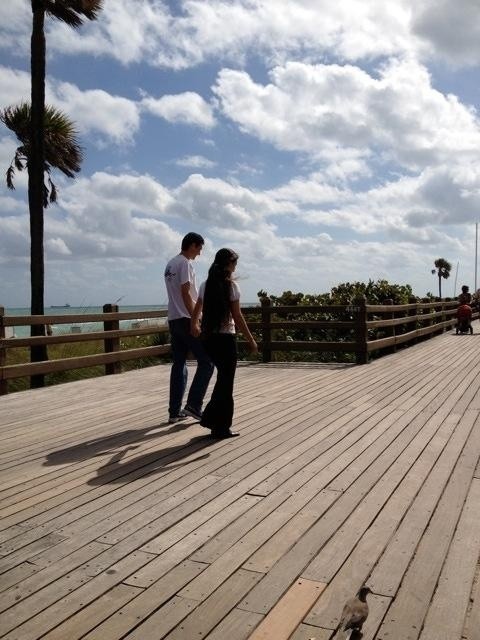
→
[169,405,202,423]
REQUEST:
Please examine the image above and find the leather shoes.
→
[200,422,240,437]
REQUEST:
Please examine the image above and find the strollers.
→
[455,304,473,335]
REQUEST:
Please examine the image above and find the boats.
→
[51,304,70,308]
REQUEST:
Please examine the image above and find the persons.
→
[191,246,259,440]
[458,284,473,305]
[163,232,210,424]
